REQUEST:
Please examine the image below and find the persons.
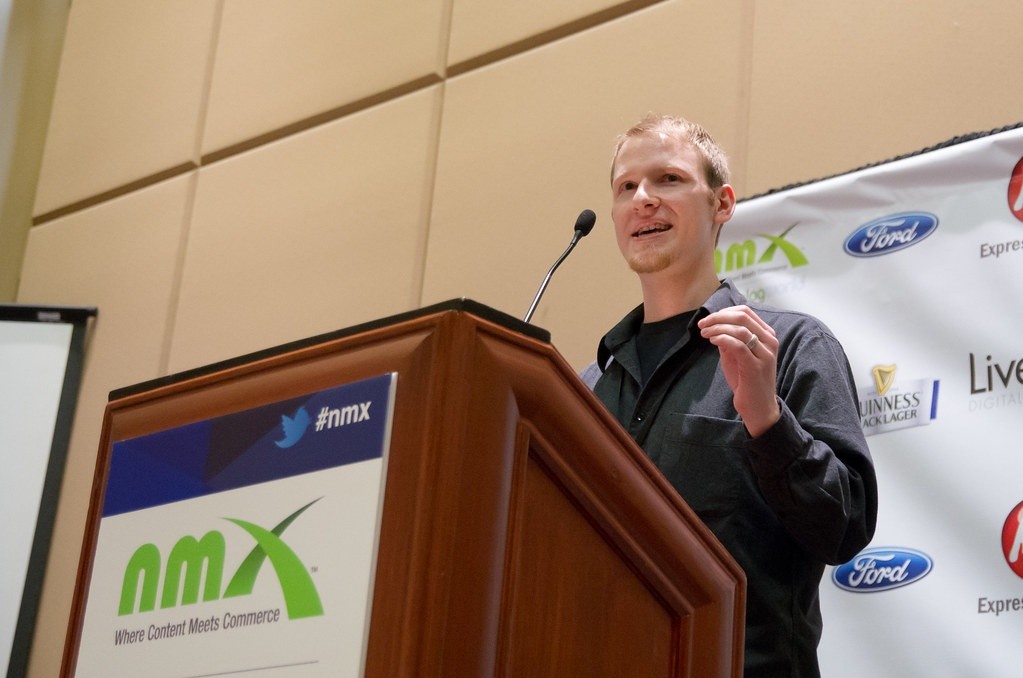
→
[578,117,878,678]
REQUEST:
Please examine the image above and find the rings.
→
[745,334,758,351]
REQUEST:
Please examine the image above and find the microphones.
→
[524,210,597,324]
